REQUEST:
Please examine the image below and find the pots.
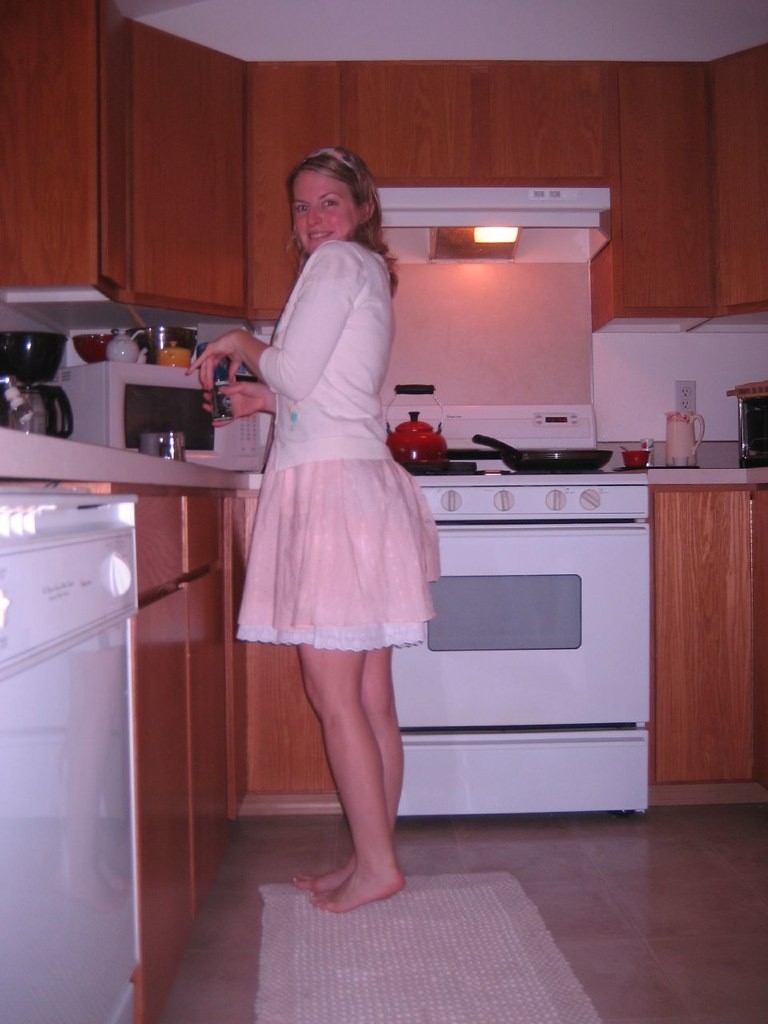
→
[472,434,613,471]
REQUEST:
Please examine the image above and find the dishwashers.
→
[1,490,140,1024]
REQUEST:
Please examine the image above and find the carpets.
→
[254,871,602,1024]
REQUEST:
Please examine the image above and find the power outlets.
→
[675,379,696,414]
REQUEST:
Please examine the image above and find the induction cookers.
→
[382,405,649,519]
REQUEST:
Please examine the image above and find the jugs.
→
[8,384,73,438]
[665,410,705,465]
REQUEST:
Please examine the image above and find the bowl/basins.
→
[127,326,198,364]
[0,331,67,380]
[621,450,651,466]
[74,333,113,364]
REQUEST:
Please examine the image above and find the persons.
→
[186,146,440,914]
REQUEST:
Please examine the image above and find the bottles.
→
[639,439,655,467]
[106,330,139,362]
[4,381,34,424]
[212,367,234,420]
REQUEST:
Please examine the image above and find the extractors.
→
[374,178,610,268]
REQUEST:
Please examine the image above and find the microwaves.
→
[62,365,260,470]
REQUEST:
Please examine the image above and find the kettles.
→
[386,384,450,467]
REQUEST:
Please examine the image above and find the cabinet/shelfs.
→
[647,484,768,808]
[0,2,768,325]
[223,489,345,819]
[81,487,233,1024]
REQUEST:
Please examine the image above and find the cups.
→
[157,340,192,368]
[139,432,185,461]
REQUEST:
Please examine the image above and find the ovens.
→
[390,522,648,815]
